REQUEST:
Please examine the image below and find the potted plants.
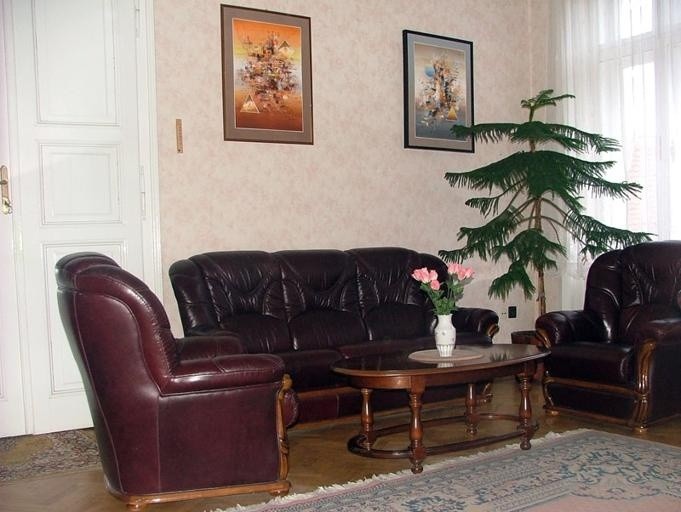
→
[437,90,658,384]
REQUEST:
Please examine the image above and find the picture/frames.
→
[220,3,314,145]
[403,28,475,154]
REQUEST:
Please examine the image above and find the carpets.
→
[204,428,681,512]
[0,430,104,488]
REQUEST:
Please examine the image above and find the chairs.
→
[536,240,681,435]
[54,251,298,512]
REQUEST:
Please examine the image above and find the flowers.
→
[411,263,476,316]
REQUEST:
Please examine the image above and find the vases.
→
[435,314,457,358]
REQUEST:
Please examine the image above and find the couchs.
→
[168,247,499,434]
